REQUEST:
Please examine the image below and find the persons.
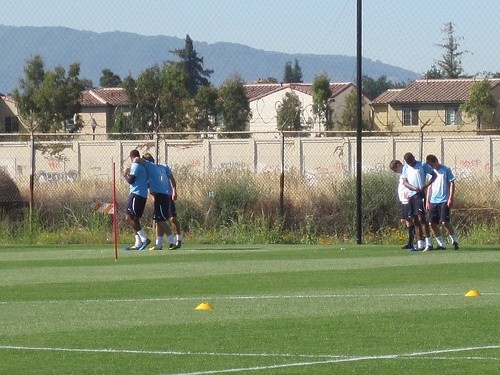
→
[389,160,425,249]
[143,152,181,248]
[133,152,177,250]
[402,152,433,252]
[123,150,150,251]
[425,155,458,250]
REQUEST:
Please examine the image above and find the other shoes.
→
[169,241,182,250]
[149,245,162,250]
[433,246,446,250]
[401,245,433,252]
[125,246,139,250]
[453,242,459,250]
[139,239,151,250]
